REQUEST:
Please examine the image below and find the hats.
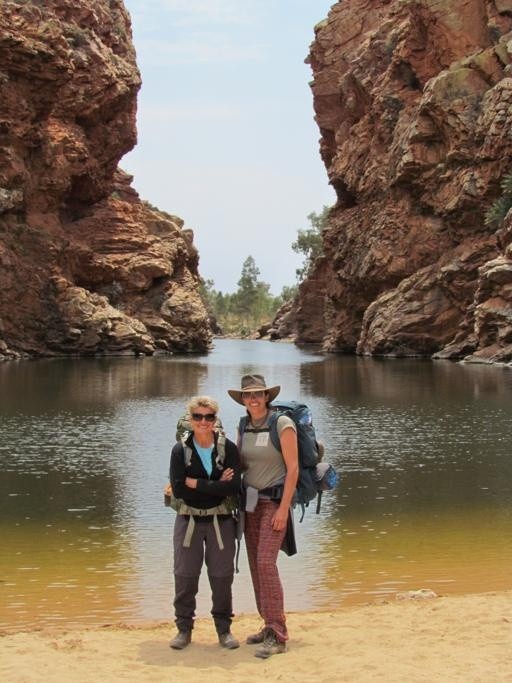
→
[227,374,280,405]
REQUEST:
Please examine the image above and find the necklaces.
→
[250,411,268,428]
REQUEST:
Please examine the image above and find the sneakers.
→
[171,630,191,649]
[251,628,286,657]
[246,627,264,643]
[218,630,240,648]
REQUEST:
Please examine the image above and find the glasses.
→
[242,392,263,398]
[192,413,215,421]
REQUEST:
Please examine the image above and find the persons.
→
[167,392,243,651]
[228,372,301,659]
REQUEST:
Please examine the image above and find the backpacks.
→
[238,400,336,500]
[164,413,237,516]
